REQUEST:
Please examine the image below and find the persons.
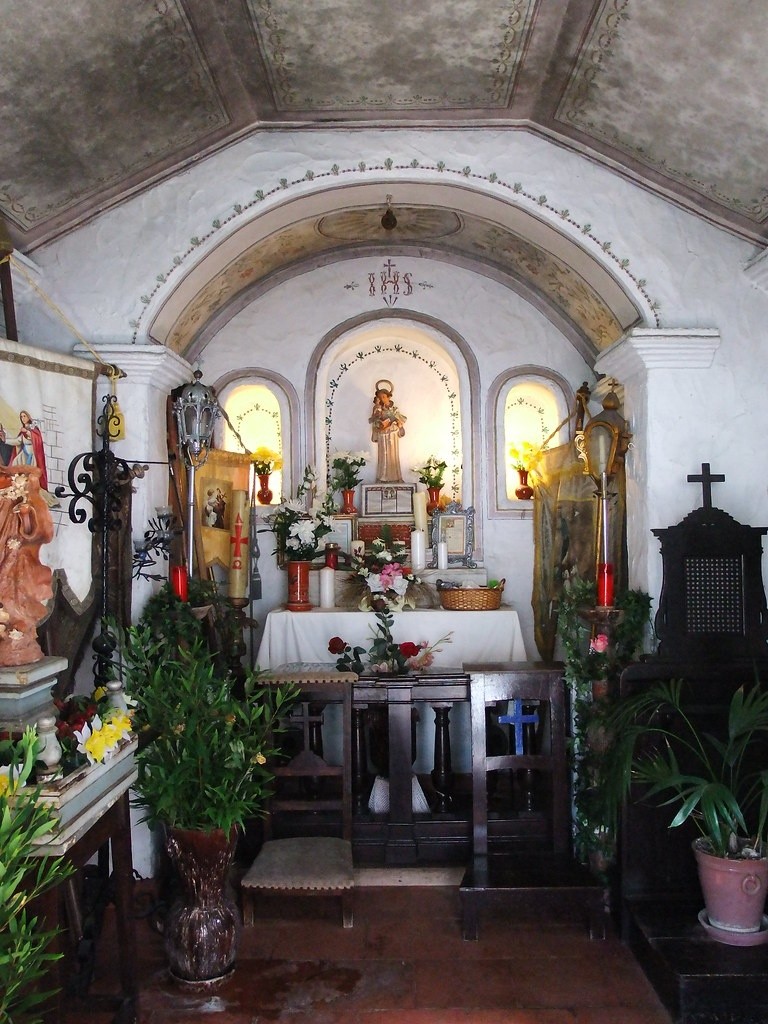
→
[369,390,407,484]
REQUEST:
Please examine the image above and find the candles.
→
[412,492,430,548]
[227,489,250,598]
[170,564,188,604]
[596,562,614,607]
[316,526,448,611]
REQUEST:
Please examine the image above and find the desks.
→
[233,663,568,867]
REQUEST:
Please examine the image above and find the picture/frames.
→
[426,501,480,570]
[276,513,359,570]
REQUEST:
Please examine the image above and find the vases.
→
[340,489,358,515]
[256,472,272,505]
[515,470,533,500]
[105,821,302,995]
[282,559,315,611]
[428,487,444,516]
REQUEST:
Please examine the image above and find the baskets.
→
[437,586,504,610]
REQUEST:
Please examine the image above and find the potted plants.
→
[584,678,768,946]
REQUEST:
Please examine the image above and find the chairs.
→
[241,671,359,930]
[459,663,607,942]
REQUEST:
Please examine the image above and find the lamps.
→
[173,370,220,578]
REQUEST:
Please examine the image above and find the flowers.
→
[326,446,371,490]
[249,446,283,474]
[508,439,542,470]
[555,575,652,843]
[0,684,135,820]
[326,598,457,672]
[410,454,447,488]
[256,465,341,562]
